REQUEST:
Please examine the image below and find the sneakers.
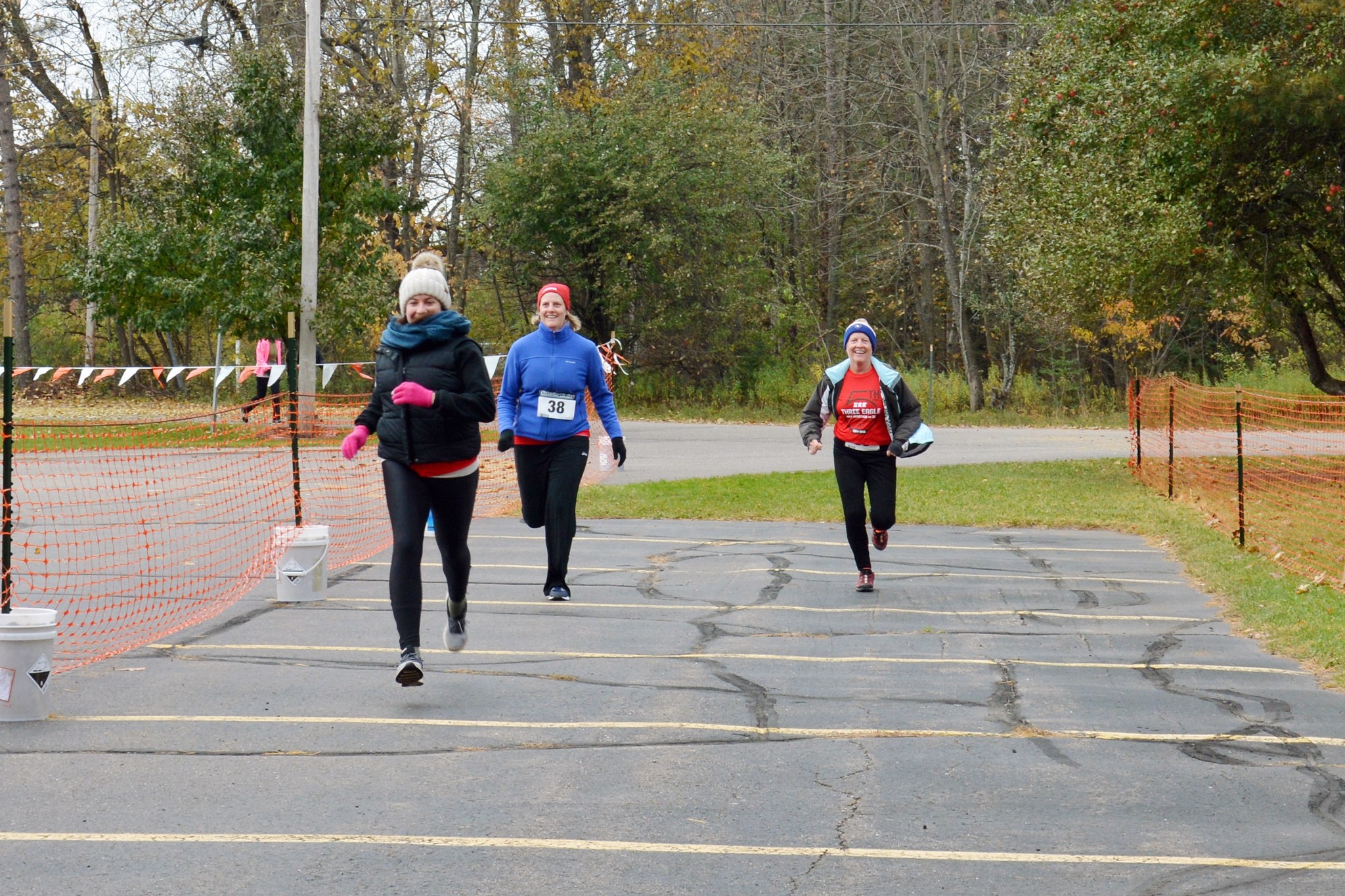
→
[548,588,570,600]
[396,646,425,687]
[442,592,468,652]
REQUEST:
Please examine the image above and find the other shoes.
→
[856,571,875,592]
[872,527,888,550]
[241,407,249,423]
[272,419,282,424]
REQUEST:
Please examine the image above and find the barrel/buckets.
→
[0,606,58,722]
[599,436,626,471]
[270,524,330,601]
[423,509,436,537]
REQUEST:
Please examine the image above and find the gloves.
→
[612,437,626,466]
[390,381,433,407]
[341,425,369,461]
[498,429,515,452]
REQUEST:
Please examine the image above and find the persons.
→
[799,319,935,592]
[498,283,626,602]
[241,338,325,423]
[341,251,496,687]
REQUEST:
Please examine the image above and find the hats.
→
[399,251,451,316]
[537,283,570,310]
[843,318,877,354]
